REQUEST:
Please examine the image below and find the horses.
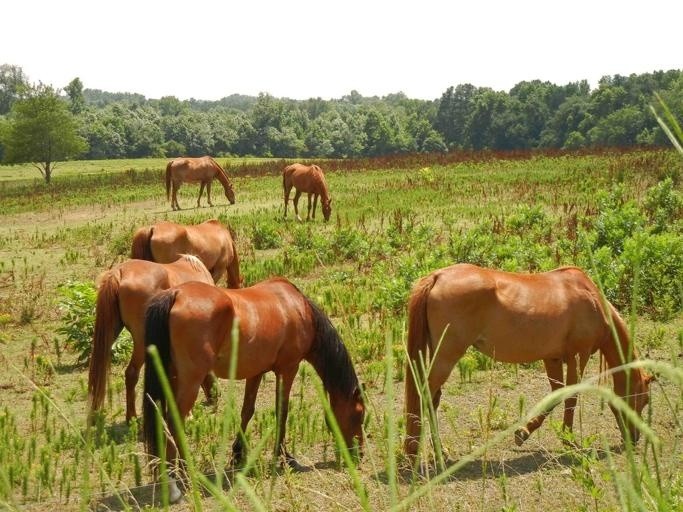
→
[283,163,332,222]
[131,219,242,288]
[405,263,656,465]
[87,253,221,424]
[143,277,366,503]
[166,156,236,211]
